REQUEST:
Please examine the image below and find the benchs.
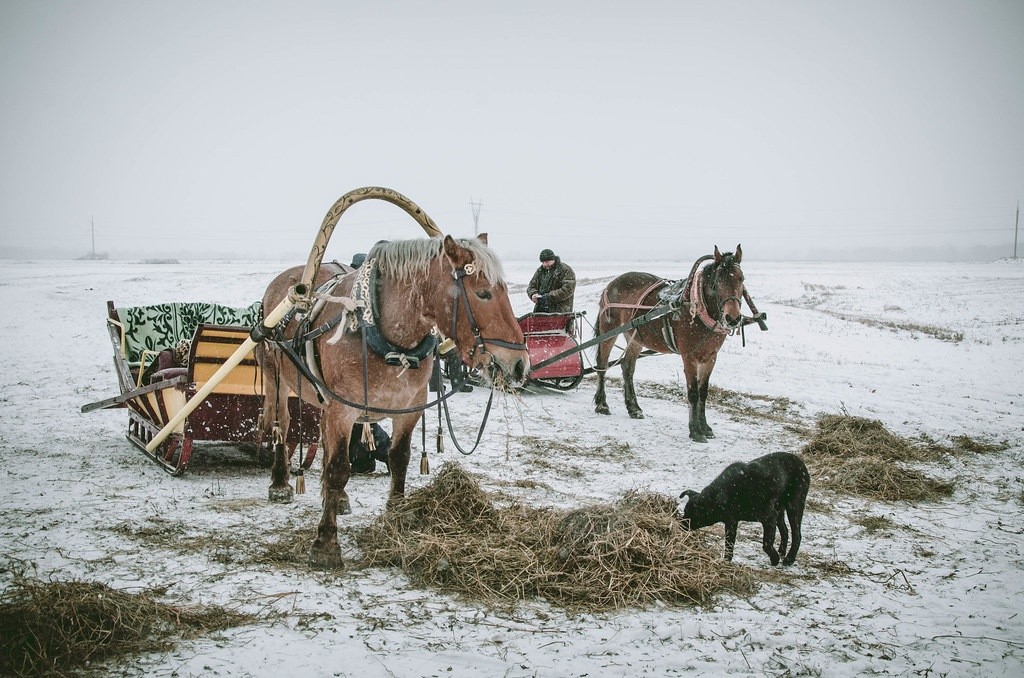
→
[107,301,262,382]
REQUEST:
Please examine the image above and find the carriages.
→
[442,243,768,443]
[81,186,531,573]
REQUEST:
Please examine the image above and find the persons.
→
[350,424,390,475]
[526,249,576,333]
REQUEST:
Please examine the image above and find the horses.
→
[593,244,746,445]
[256,233,532,572]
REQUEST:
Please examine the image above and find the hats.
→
[540,249,556,262]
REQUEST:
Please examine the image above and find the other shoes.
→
[430,385,446,391]
[384,439,393,475]
[564,377,578,383]
[349,461,376,476]
[452,382,473,392]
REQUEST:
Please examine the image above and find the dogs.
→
[680,452,811,566]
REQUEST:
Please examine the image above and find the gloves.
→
[538,293,552,307]
